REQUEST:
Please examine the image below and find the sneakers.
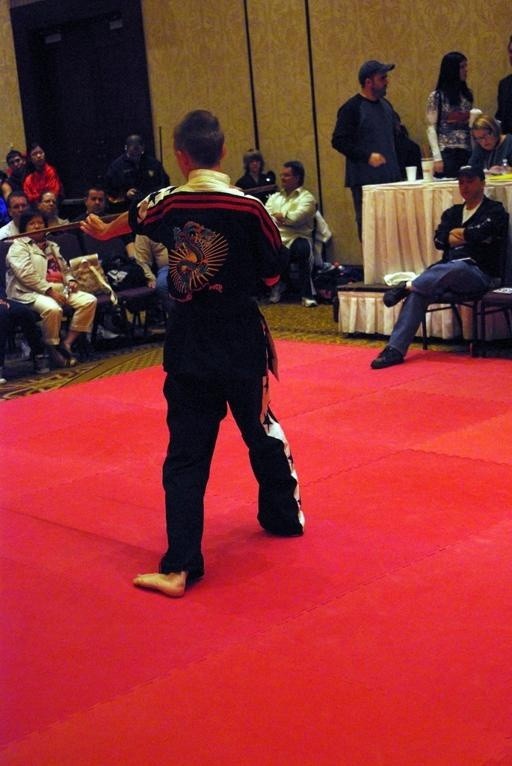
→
[370,344,405,370]
[32,342,78,374]
[3,352,34,370]
[269,281,288,304]
[301,296,319,308]
[382,280,412,308]
[147,326,167,340]
[20,342,31,361]
[0,366,8,384]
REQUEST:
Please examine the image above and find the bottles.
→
[500,159,510,175]
[62,285,71,299]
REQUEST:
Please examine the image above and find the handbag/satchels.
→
[104,253,147,292]
[68,253,118,305]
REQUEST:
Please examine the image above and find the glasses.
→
[473,130,492,142]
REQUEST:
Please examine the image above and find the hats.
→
[455,164,485,178]
[358,60,396,84]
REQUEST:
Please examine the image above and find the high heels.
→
[95,324,120,344]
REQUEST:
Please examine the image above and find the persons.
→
[0,136,168,385]
[424,40,511,181]
[371,165,511,368]
[236,148,346,307]
[79,109,306,597]
[331,59,402,244]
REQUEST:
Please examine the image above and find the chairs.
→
[418,208,510,357]
[0,169,279,374]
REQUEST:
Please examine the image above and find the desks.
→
[358,174,511,340]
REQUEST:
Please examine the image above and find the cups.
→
[467,108,483,128]
[420,158,436,182]
[405,166,418,182]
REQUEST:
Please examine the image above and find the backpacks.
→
[312,263,365,322]
